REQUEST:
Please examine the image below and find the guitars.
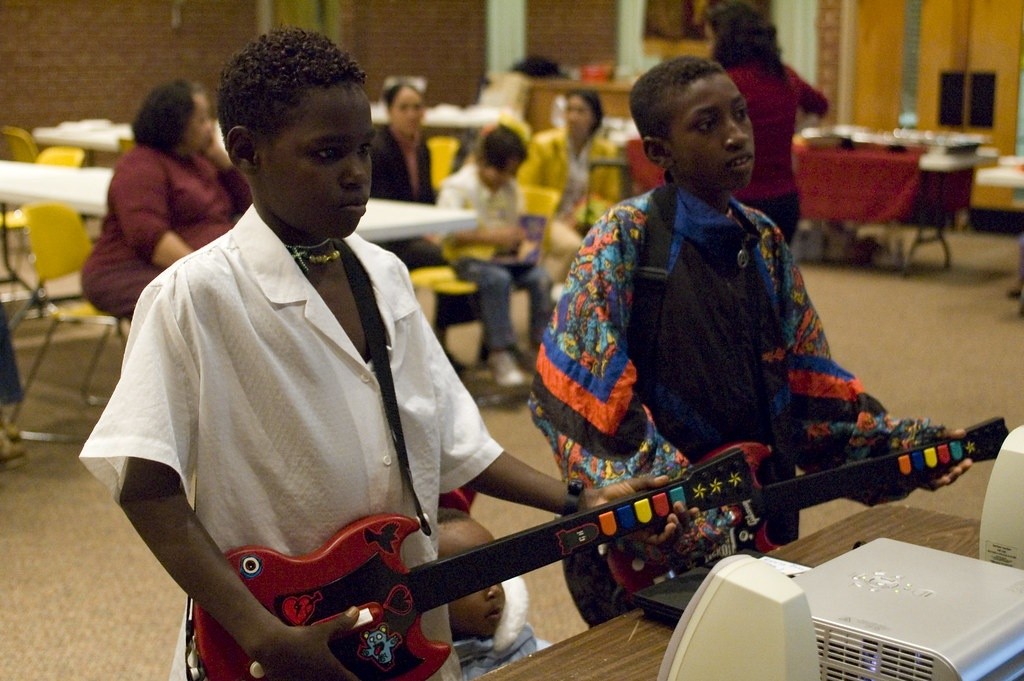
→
[596,417,1008,607]
[187,448,757,680]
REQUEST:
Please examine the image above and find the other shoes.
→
[0,433,27,471]
[490,356,523,389]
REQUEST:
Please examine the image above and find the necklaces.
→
[284,238,342,272]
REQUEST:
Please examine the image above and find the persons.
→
[80,79,255,321]
[525,51,973,628]
[434,505,536,680]
[701,0,829,254]
[77,20,703,681]
[367,71,629,388]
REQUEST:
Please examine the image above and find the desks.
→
[474,504,983,681]
[0,159,478,264]
[790,144,998,278]
[525,78,633,135]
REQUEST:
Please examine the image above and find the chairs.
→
[0,126,560,445]
